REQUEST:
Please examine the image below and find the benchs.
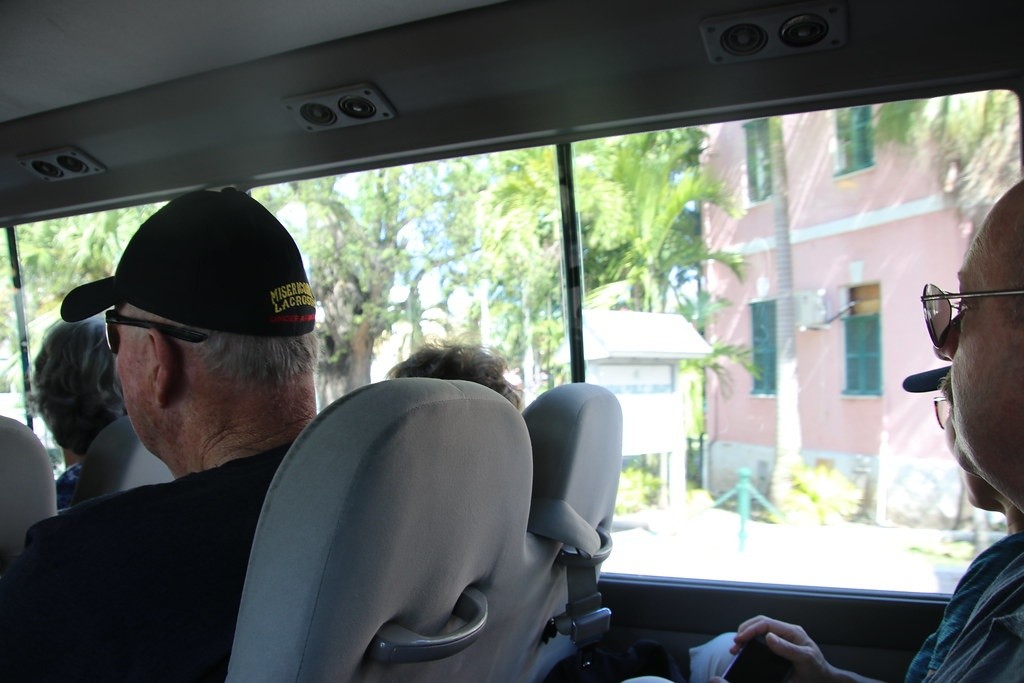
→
[225,381,622,683]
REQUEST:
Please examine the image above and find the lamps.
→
[796,301,857,332]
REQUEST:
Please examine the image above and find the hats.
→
[901,366,949,392]
[62,189,316,338]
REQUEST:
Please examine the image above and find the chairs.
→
[0,415,59,589]
[82,415,176,505]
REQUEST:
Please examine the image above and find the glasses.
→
[919,280,1024,348]
[105,308,210,353]
[932,397,953,429]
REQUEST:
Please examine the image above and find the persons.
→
[387,340,524,410]
[625,181,1024,683]
[0,187,313,683]
